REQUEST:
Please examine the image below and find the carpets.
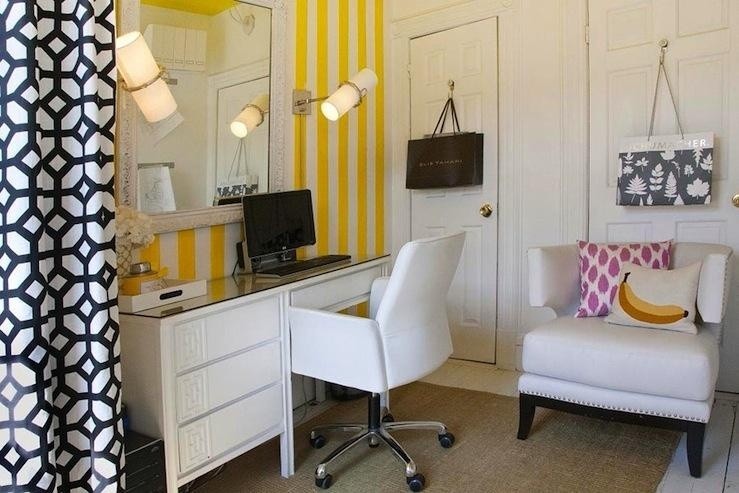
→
[183,380,681,491]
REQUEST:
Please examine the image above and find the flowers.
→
[113,206,156,275]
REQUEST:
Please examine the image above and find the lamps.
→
[115,31,178,126]
[230,91,269,137]
[293,67,382,121]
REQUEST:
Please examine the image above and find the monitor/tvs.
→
[242,189,316,267]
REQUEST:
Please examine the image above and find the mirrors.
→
[118,0,296,237]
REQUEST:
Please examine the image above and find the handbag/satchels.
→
[212,139,259,206]
[405,98,484,190]
[616,63,715,207]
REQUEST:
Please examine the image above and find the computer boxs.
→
[124,430,168,493]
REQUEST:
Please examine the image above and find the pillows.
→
[576,237,701,337]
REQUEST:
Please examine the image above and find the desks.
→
[117,251,390,493]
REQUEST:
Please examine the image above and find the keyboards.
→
[255,254,351,280]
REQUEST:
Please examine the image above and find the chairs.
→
[288,229,469,492]
[514,240,733,481]
